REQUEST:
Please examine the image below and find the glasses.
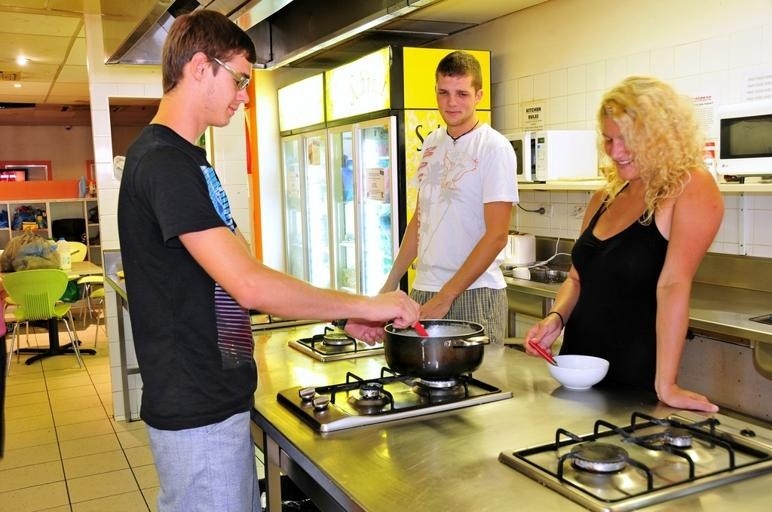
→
[214,57,249,90]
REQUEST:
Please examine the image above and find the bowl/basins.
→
[546,353,613,394]
[534,264,567,283]
[512,267,532,281]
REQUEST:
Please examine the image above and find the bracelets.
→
[331,317,347,332]
[546,311,564,330]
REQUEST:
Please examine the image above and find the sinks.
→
[748,313,772,381]
[502,269,573,319]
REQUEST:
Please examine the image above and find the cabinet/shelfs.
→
[0,197,105,270]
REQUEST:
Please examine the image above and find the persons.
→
[522,75,725,414]
[378,50,520,347]
[116,9,422,511]
[0,278,9,460]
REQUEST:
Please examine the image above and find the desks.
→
[251,304,771,511]
[15,261,105,366]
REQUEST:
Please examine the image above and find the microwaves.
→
[500,130,600,181]
[714,109,772,179]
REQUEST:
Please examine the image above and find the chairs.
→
[91,287,107,347]
[56,241,87,319]
[3,270,84,376]
[541,203,555,217]
[77,275,104,330]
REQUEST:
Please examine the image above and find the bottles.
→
[36,214,43,229]
[79,175,87,198]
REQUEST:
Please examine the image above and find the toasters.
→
[499,230,537,268]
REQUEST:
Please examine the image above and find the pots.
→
[382,320,489,383]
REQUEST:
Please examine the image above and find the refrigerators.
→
[276,45,492,299]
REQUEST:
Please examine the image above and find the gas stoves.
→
[496,407,771,512]
[288,324,384,362]
[274,367,513,433]
